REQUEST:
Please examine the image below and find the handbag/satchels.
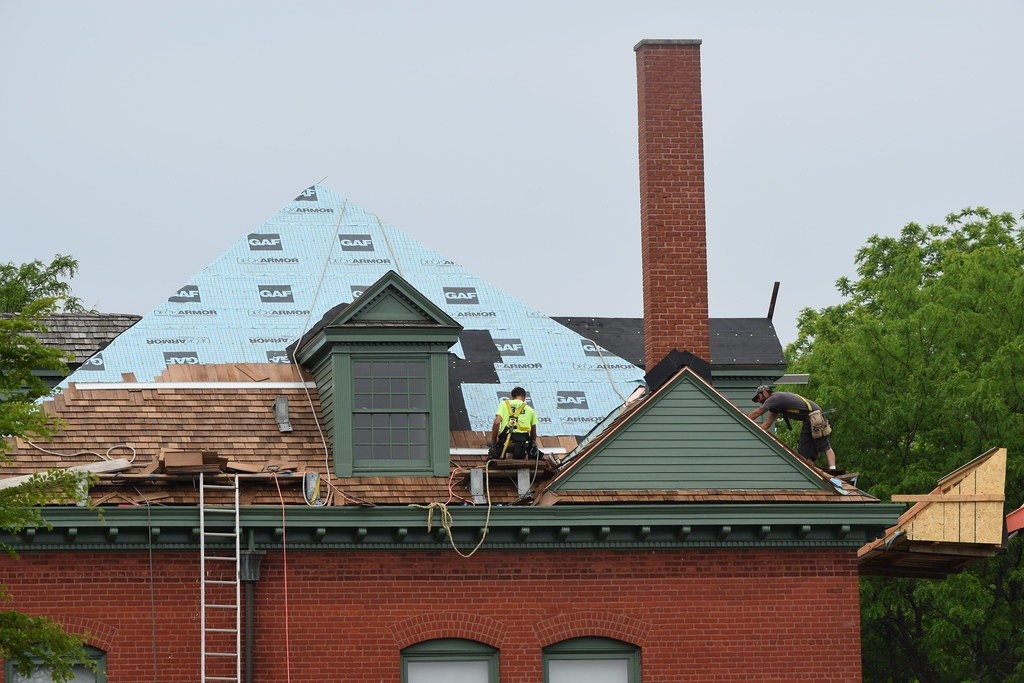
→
[809,410,831,438]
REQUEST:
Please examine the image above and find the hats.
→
[751,385,772,402]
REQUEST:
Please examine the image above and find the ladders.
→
[198,473,241,683]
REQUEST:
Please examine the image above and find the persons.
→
[748,384,836,474]
[487,387,545,461]
